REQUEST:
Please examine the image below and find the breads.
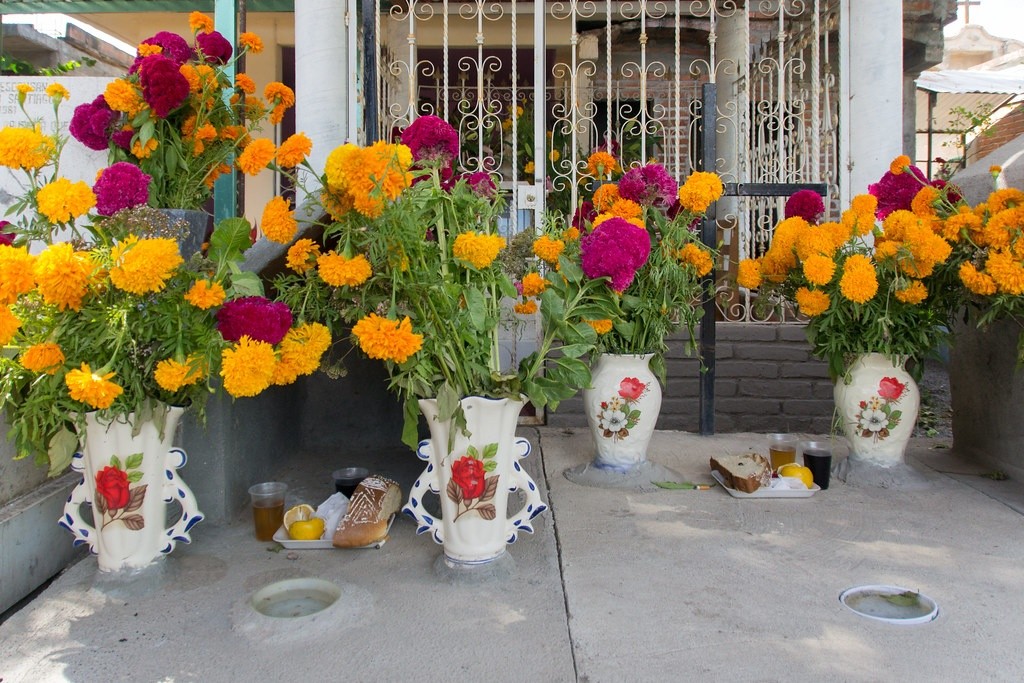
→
[709,454,771,494]
[333,474,403,548]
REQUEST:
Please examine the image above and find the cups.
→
[766,434,800,471]
[248,482,288,542]
[332,467,370,500]
[801,441,833,490]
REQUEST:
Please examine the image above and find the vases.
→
[583,353,662,469]
[56,400,206,574]
[833,352,920,469]
[402,392,549,568]
[146,209,207,279]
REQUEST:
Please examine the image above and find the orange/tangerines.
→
[283,503,324,540]
[777,462,814,490]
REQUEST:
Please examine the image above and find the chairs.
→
[683,219,739,322]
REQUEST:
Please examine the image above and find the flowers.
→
[737,155,1024,436]
[0,11,737,478]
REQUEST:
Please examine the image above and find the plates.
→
[711,470,821,498]
[272,513,396,549]
